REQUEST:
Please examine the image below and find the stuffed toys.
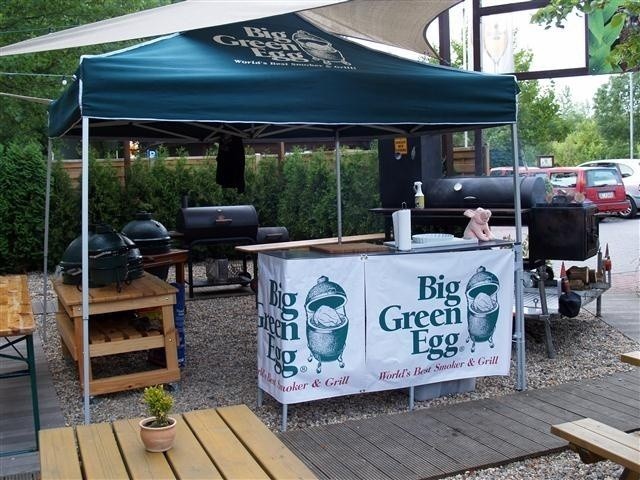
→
[464,207,495,242]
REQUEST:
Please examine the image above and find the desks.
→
[38,404,319,480]
[50,270,180,402]
[621,351,640,367]
[0,274,41,451]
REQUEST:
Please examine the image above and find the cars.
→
[576,159,640,219]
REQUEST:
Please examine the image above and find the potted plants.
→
[139,384,177,453]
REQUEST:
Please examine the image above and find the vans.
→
[481,166,630,220]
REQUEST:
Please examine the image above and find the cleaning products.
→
[413,181,424,209]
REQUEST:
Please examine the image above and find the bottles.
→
[602,256,611,270]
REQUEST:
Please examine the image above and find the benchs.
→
[550,417,640,480]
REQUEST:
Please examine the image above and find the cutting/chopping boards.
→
[383,238,478,248]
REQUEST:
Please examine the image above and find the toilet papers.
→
[391,209,411,251]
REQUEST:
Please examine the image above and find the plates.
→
[412,233,454,244]
